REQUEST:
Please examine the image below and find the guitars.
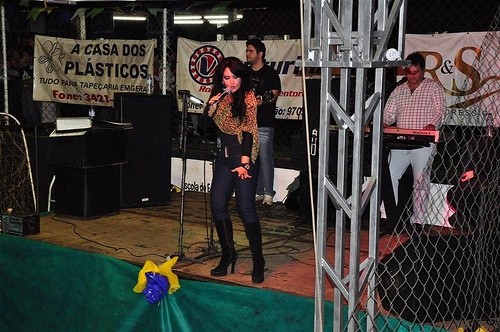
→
[258,88,276,104]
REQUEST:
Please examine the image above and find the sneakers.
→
[262,194,273,205]
[255,194,264,201]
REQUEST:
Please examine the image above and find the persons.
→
[0,31,42,130]
[198,57,265,283]
[61,26,189,117]
[447,141,500,234]
[246,37,281,205]
[371,51,444,230]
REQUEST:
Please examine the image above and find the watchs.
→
[239,163,250,171]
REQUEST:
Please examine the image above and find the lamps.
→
[172,14,202,20]
[204,14,245,25]
[112,15,148,22]
[174,19,204,24]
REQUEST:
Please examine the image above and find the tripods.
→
[159,96,222,266]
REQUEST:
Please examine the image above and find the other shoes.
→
[377,218,389,233]
[411,224,425,235]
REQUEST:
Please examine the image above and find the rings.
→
[241,173,244,176]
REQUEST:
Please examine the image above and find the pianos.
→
[325,125,442,236]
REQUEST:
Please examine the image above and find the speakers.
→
[115,93,171,208]
[55,164,122,220]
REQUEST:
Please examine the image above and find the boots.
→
[210,216,238,277]
[244,222,265,284]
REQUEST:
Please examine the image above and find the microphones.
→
[209,87,231,106]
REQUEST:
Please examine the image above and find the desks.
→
[37,122,127,220]
